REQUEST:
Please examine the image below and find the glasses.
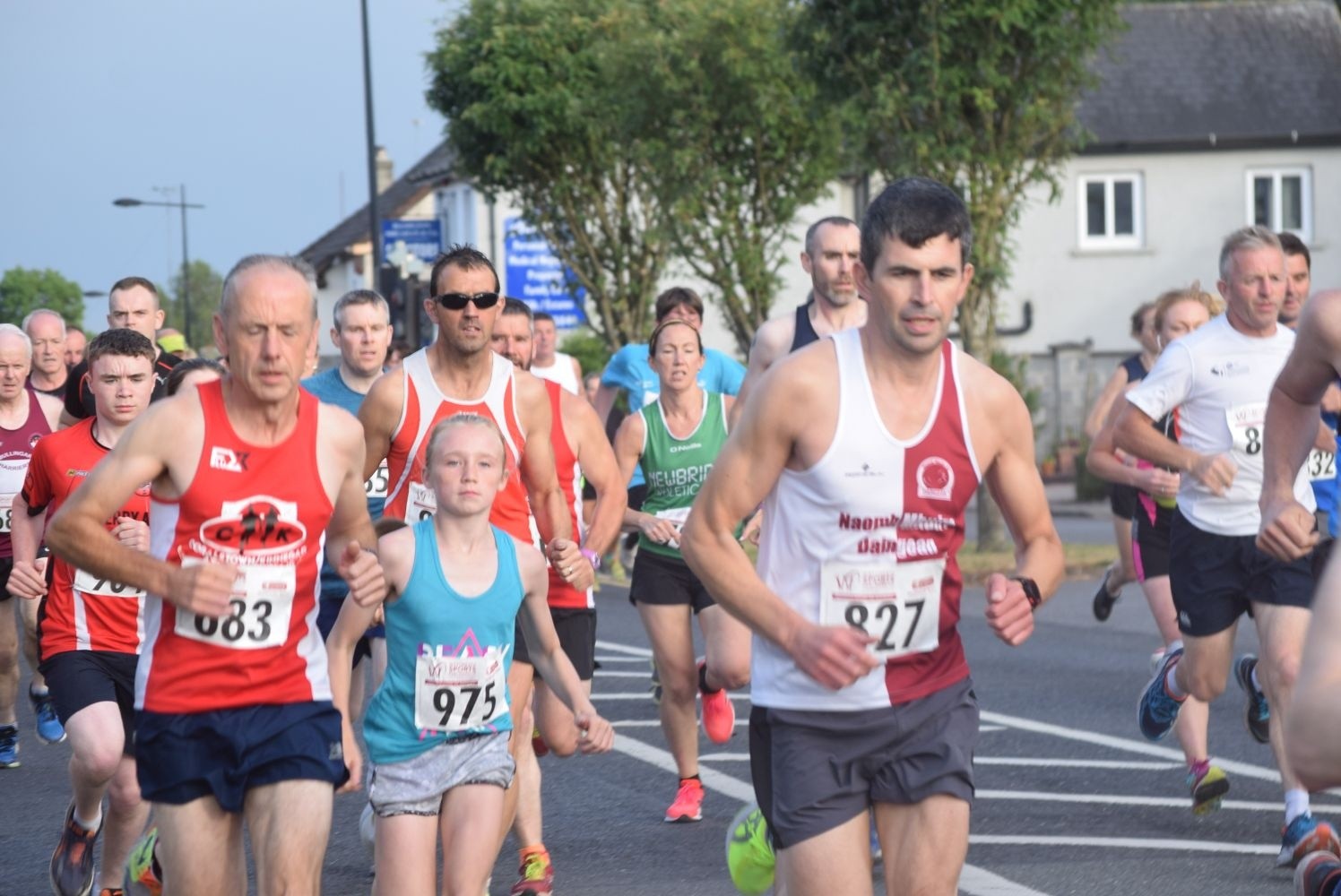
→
[432,293,498,309]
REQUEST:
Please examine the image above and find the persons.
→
[0,219,890,896]
[675,177,1069,896]
[1086,223,1340,896]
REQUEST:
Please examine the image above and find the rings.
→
[661,539,665,542]
[569,567,573,574]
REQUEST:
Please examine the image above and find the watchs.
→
[581,547,602,570]
[1009,575,1043,612]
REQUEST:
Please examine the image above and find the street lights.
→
[111,184,207,349]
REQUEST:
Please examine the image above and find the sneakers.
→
[1274,809,1341,870]
[1092,565,1122,622]
[1293,850,1341,896]
[510,844,554,896]
[1185,754,1230,816]
[27,680,68,745]
[1137,638,1190,741]
[532,721,550,757]
[694,655,735,744]
[90,872,130,896]
[1234,653,1271,744]
[48,796,104,896]
[664,779,706,823]
[0,721,21,767]
